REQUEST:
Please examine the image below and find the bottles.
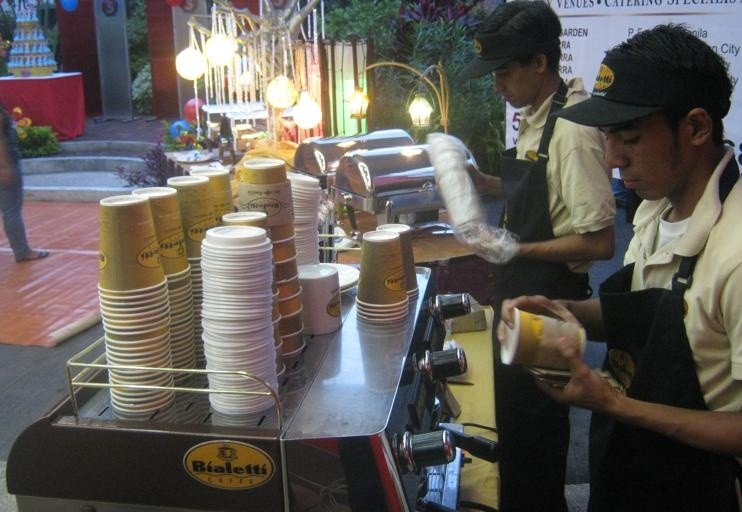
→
[7,8,57,68]
[217,113,236,166]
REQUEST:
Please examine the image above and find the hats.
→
[460,35,560,79]
[550,63,733,127]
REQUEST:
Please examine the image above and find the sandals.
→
[17,251,48,262]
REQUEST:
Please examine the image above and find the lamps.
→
[173,0,447,131]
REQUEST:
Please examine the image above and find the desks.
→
[0,71,86,140]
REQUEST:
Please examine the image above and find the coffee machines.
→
[2,264,502,511]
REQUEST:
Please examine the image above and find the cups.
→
[352,224,417,325]
[430,133,487,238]
[292,258,342,336]
[499,305,589,368]
[94,154,327,430]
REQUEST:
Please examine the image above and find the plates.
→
[176,154,212,164]
[317,263,360,295]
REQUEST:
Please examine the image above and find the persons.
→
[0,103,50,262]
[498,23,741,512]
[454,2,617,512]
